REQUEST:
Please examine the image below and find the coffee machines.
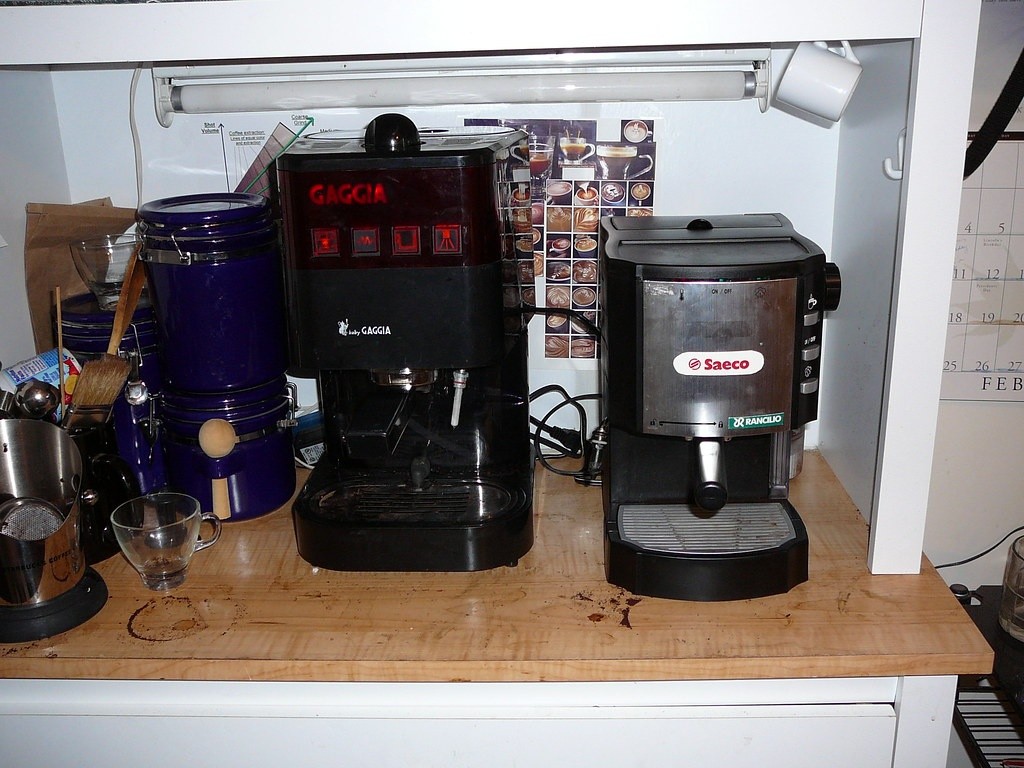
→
[276,113,534,572]
[599,213,842,602]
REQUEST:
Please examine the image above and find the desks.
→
[1,410,995,766]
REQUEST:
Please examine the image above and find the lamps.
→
[148,47,778,128]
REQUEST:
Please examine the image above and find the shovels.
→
[107,237,149,355]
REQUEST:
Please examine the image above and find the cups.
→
[110,492,222,592]
[597,145,653,180]
[529,136,555,178]
[998,535,1024,644]
[560,137,595,163]
[52,193,299,522]
[624,122,652,143]
[547,183,571,204]
[776,39,863,122]
[576,187,598,206]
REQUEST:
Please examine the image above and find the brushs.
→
[64,359,133,429]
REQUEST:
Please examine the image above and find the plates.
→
[543,181,650,358]
